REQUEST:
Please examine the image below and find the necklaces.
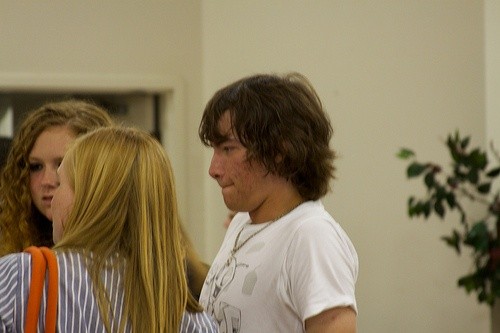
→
[225,201,308,268]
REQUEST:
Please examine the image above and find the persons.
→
[196,72,360,333]
[0,101,113,256]
[0,126,222,333]
